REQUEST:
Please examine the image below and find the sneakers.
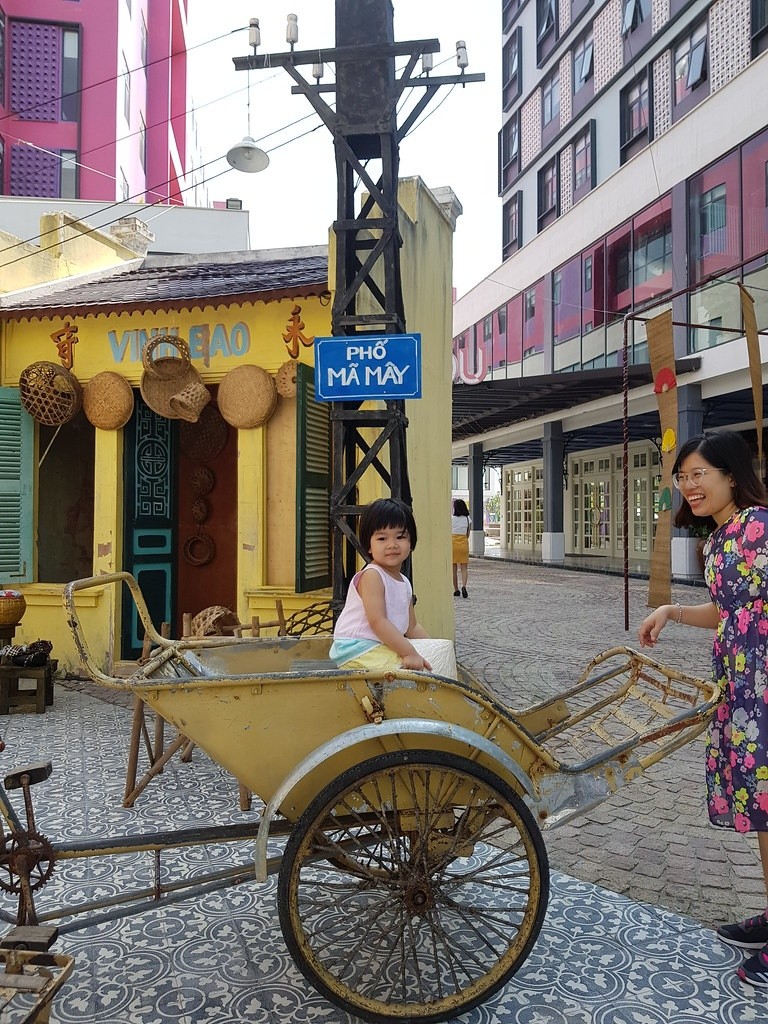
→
[738,941,768,987]
[717,907,768,949]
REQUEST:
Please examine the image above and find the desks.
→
[0,624,22,648]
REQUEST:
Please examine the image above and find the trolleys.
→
[1,573,723,1022]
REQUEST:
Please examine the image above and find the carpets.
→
[564,553,608,557]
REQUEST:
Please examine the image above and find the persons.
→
[451,498,472,598]
[639,429,768,990]
[327,500,433,677]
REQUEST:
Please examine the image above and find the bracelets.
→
[674,602,682,625]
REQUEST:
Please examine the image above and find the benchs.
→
[290,660,337,671]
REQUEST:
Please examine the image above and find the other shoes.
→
[454,590,460,596]
[462,587,468,598]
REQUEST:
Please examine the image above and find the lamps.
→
[226,197,242,210]
[227,56,269,173]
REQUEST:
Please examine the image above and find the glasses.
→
[672,468,723,489]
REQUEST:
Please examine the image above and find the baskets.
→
[178,405,228,462]
[0,596,26,624]
[18,361,83,426]
[190,466,214,495]
[83,371,134,431]
[217,364,278,429]
[192,500,207,523]
[168,382,212,423]
[274,360,302,398]
[140,356,204,419]
[191,605,232,636]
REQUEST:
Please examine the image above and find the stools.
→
[0,660,59,716]
